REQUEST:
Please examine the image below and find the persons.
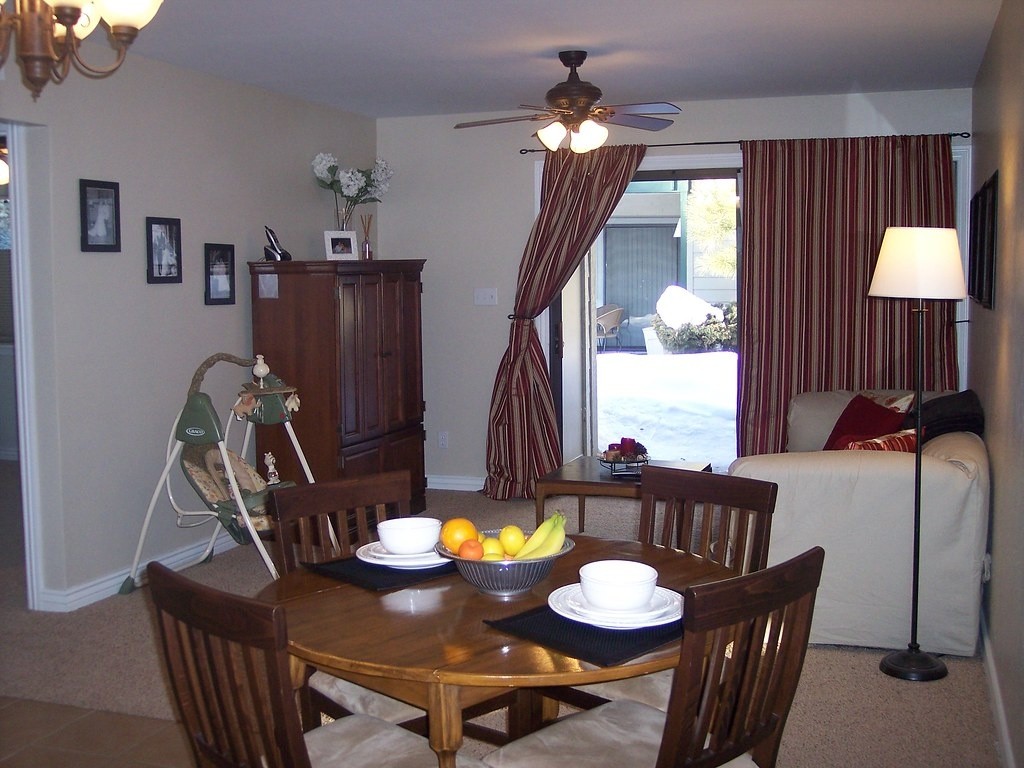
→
[153,232,175,275]
[335,242,345,252]
[88,200,109,237]
[213,258,229,291]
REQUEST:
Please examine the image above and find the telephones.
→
[264,225,292,261]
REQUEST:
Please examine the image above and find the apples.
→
[459,539,483,560]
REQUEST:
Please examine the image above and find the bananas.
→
[513,510,566,560]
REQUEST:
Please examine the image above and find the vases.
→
[334,208,352,231]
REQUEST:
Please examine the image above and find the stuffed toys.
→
[285,393,300,412]
[230,392,262,419]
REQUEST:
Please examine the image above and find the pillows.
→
[848,428,928,455]
[885,393,915,413]
[824,396,903,448]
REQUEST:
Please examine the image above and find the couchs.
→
[732,390,990,659]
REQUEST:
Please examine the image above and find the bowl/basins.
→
[377,517,443,556]
[579,559,658,614]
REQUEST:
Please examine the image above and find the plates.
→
[355,541,455,568]
[548,580,685,630]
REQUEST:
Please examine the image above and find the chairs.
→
[120,352,340,592]
[598,305,623,351]
[533,463,779,730]
[482,546,824,768]
[268,473,515,747]
[147,560,477,767]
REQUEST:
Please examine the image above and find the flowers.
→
[314,152,394,212]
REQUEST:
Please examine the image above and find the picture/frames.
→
[966,170,999,310]
[204,242,236,305]
[79,179,121,253]
[324,230,359,260]
[146,216,183,283]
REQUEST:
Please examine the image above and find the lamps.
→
[536,121,608,154]
[1,0,162,103]
[868,227,967,682]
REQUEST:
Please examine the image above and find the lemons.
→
[477,525,525,560]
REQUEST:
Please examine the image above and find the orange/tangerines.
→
[440,518,478,554]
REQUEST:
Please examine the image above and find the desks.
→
[254,534,741,768]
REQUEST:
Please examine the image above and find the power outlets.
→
[438,431,448,448]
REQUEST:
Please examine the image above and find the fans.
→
[455,52,682,131]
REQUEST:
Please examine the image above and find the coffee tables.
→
[535,455,713,541]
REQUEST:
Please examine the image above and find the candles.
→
[609,444,621,452]
[620,437,635,454]
[606,451,619,460]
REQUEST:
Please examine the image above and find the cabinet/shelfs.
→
[246,260,424,548]
